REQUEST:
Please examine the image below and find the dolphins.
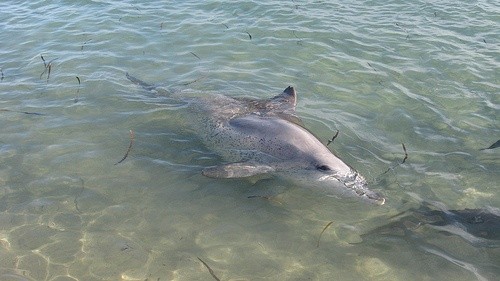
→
[348,203,500,247]
[122,72,385,205]
